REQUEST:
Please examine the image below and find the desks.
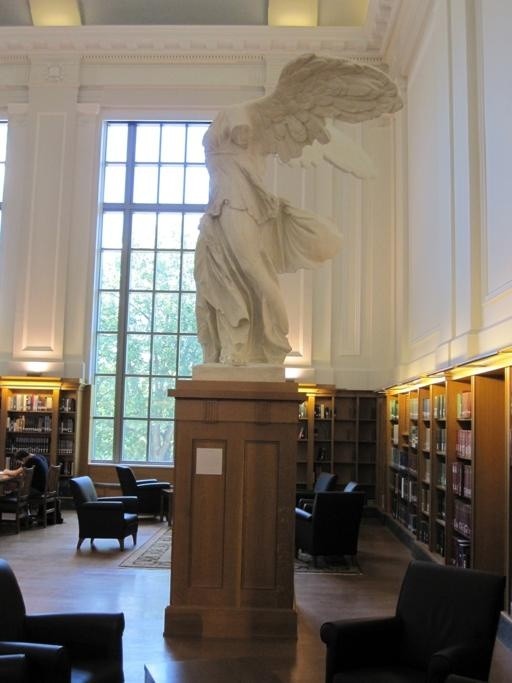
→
[158,488,175,526]
[141,654,283,682]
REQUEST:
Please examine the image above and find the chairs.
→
[1,558,126,683]
[1,464,37,533]
[295,492,363,567]
[114,465,172,520]
[296,470,338,507]
[297,482,361,512]
[0,652,30,683]
[67,475,141,552]
[34,461,64,528]
[318,559,507,682]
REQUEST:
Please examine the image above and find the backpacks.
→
[36,497,64,526]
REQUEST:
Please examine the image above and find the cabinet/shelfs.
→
[382,372,508,571]
[296,387,379,506]
[1,374,82,503]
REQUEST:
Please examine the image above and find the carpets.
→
[118,519,365,577]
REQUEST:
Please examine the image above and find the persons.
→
[192,48,406,366]
[0,448,48,517]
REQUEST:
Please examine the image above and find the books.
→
[389,388,474,572]
[5,394,75,496]
[298,399,337,482]
